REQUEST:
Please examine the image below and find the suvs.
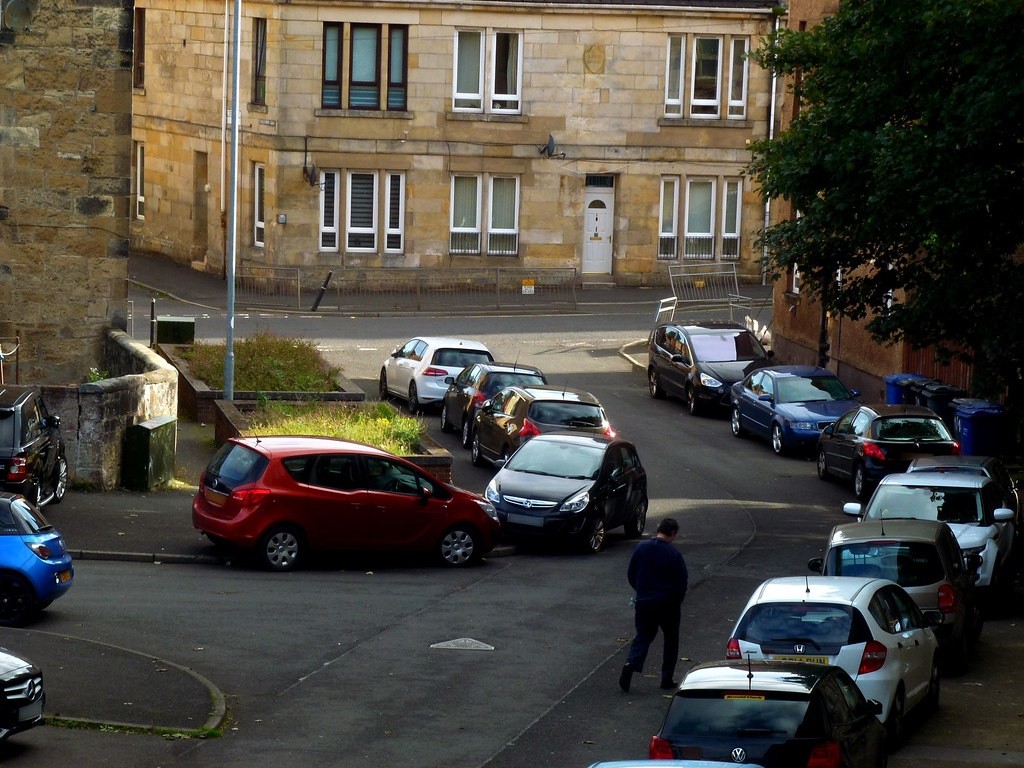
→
[0,383,72,510]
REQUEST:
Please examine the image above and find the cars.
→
[440,360,560,447]
[813,400,965,500]
[728,364,864,456]
[646,318,776,415]
[807,515,983,660]
[482,431,651,553]
[1,492,75,613]
[468,385,619,473]
[904,453,1020,516]
[378,335,495,412]
[192,436,502,574]
[0,647,43,742]
[839,473,1016,597]
[651,662,888,768]
[727,574,945,738]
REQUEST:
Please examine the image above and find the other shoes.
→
[619,663,632,690]
[660,680,678,689]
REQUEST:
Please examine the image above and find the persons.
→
[619,518,688,691]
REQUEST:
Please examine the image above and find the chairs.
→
[842,547,881,577]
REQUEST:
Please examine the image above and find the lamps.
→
[539,136,559,159]
[301,164,318,187]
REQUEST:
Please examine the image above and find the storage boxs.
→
[522,285,534,295]
[695,280,706,288]
[521,279,534,285]
